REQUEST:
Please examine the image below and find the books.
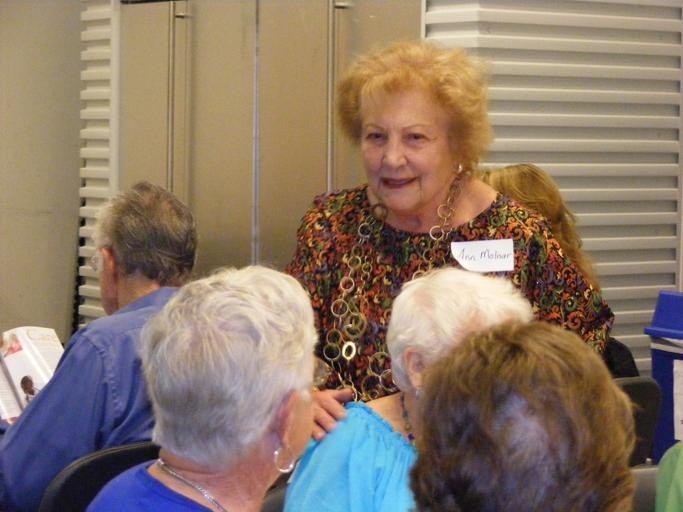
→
[1,326,65,427]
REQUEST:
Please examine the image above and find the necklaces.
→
[395,393,418,449]
[324,169,469,409]
[155,458,233,512]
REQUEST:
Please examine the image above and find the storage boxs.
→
[644,292,683,463]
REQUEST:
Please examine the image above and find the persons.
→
[282,266,534,512]
[0,182,199,512]
[479,163,603,296]
[411,325,638,512]
[289,40,614,435]
[84,265,318,512]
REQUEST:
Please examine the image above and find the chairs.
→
[39,441,161,512]
[624,468,657,512]
[618,379,660,467]
